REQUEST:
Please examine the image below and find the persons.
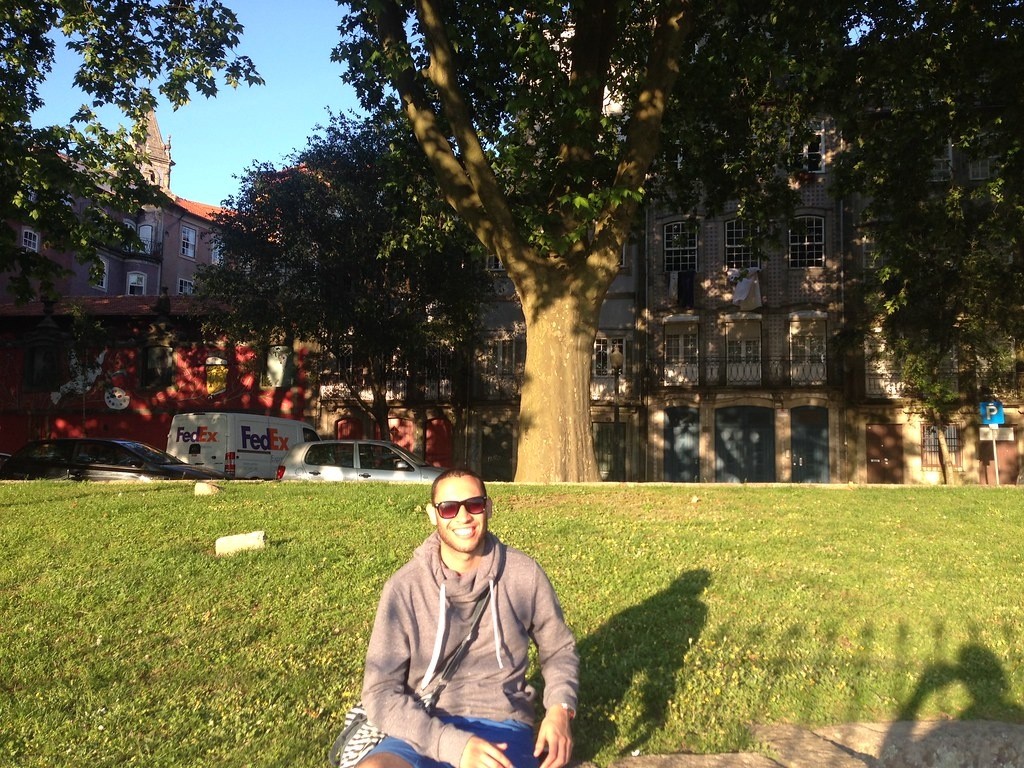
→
[352,469,581,768]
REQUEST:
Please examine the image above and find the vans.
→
[167,412,324,484]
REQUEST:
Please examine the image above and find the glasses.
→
[433,496,491,520]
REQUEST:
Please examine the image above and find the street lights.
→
[606,342,626,481]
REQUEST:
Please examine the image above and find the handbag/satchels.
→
[327,687,440,768]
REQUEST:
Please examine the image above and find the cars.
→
[277,438,454,485]
[0,438,234,481]
